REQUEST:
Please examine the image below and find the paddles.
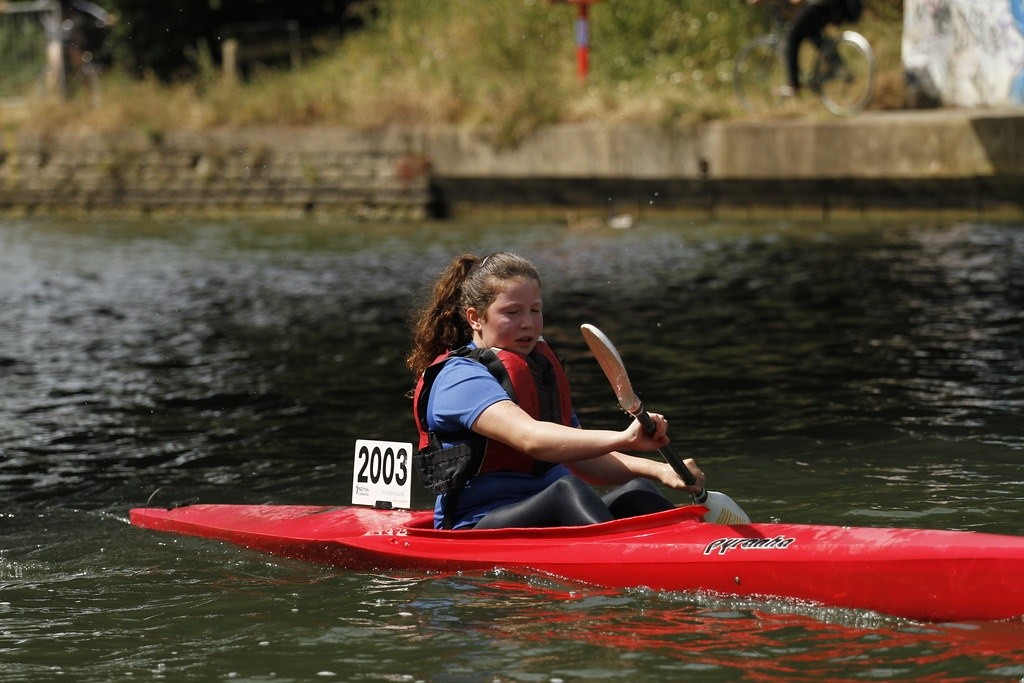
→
[579,320,753,524]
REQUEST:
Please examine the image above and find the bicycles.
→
[734,4,877,116]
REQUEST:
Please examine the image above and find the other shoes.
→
[779,84,800,97]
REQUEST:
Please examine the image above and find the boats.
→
[127,505,1024,623]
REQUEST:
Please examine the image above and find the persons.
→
[771,0,863,99]
[407,251,705,531]
